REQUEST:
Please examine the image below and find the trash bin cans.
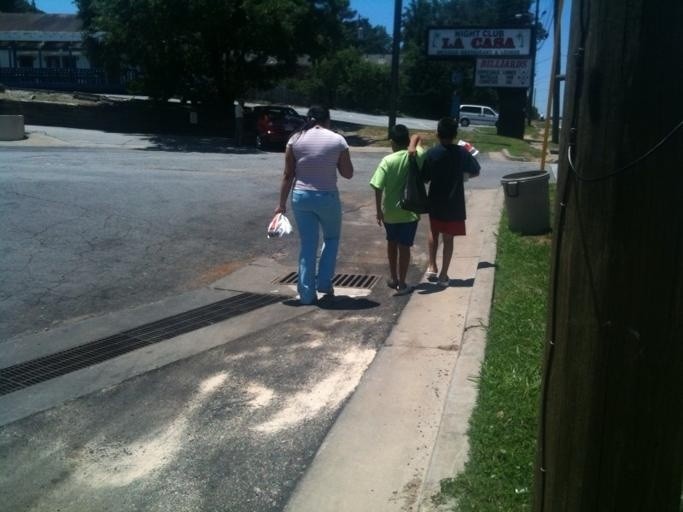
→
[500,170,553,236]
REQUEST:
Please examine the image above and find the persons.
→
[418,117,481,288]
[234,98,248,144]
[256,112,274,148]
[274,104,354,306]
[370,124,425,295]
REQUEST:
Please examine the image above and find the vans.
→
[458,104,499,127]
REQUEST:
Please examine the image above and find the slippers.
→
[438,276,450,287]
[425,271,438,282]
[385,279,399,289]
[396,284,410,293]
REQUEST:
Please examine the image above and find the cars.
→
[243,106,307,152]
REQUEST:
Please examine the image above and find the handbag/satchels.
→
[400,154,432,214]
